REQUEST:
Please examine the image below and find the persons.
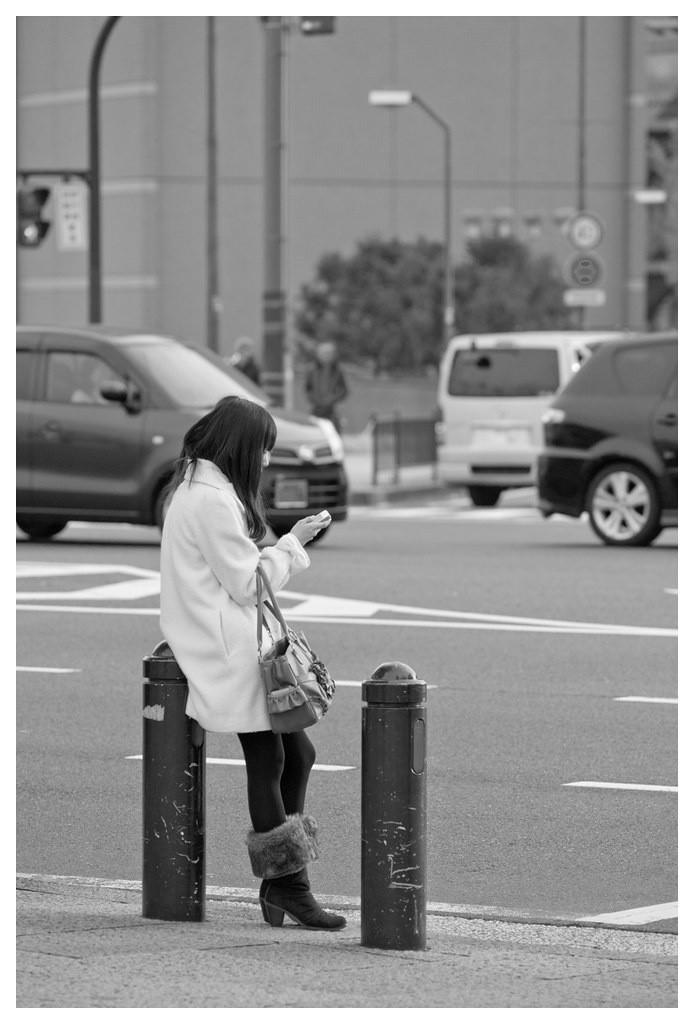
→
[306,342,349,433]
[158,396,346,930]
[230,336,261,388]
[71,361,112,406]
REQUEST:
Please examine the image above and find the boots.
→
[248,815,346,929]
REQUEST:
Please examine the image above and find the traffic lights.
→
[16,185,50,246]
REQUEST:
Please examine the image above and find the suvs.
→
[16,322,350,551]
[534,334,677,550]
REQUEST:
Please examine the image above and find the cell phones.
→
[308,509,330,523]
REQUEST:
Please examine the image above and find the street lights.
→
[369,86,455,349]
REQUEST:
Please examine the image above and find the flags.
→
[55,182,91,253]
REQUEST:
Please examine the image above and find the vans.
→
[435,329,615,505]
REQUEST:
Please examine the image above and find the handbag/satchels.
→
[256,563,337,735]
